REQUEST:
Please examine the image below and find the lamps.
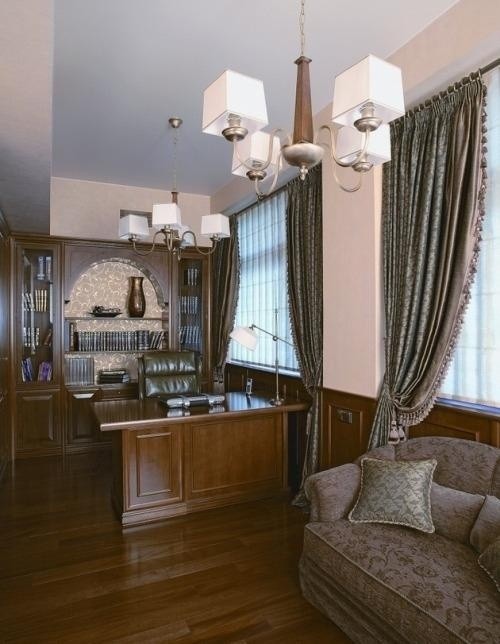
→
[118,118,231,261]
[201,0,406,202]
[229,325,293,406]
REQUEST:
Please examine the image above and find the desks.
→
[89,392,309,528]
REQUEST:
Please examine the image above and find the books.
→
[24,288,55,383]
[178,267,202,350]
[61,321,167,386]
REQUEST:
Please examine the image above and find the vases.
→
[130,276,146,318]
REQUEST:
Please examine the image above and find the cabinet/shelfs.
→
[12,240,63,459]
[69,306,169,382]
[175,253,208,390]
[65,386,133,451]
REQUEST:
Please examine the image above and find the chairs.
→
[137,352,202,399]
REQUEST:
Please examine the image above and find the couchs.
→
[298,437,500,644]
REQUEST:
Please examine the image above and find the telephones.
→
[246,378,255,395]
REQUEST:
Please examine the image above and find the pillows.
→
[348,457,500,593]
